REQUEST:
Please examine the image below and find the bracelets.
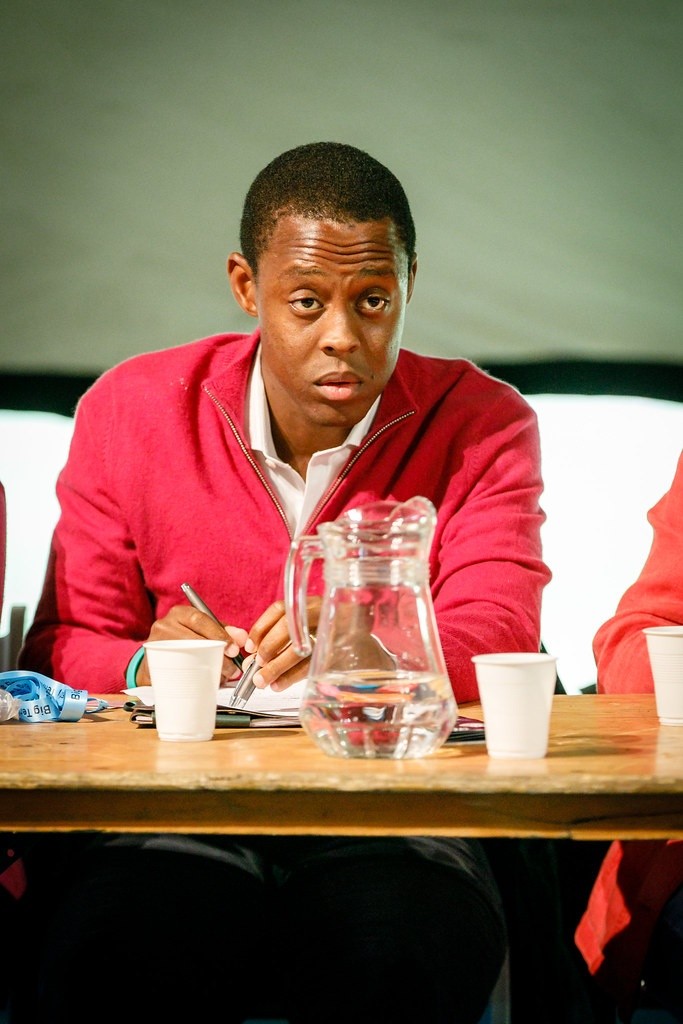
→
[125,648,146,689]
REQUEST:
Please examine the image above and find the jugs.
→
[284,498,458,759]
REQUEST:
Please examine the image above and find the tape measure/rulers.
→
[0,669,108,723]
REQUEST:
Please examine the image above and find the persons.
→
[571,452,683,1023]
[13,142,552,1023]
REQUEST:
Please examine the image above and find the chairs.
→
[520,391,683,692]
[0,411,78,666]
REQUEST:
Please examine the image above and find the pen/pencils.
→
[180,580,246,674]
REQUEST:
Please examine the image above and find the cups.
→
[642,626,683,726]
[145,639,228,741]
[471,654,559,760]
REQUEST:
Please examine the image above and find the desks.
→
[0,692,683,1024]
